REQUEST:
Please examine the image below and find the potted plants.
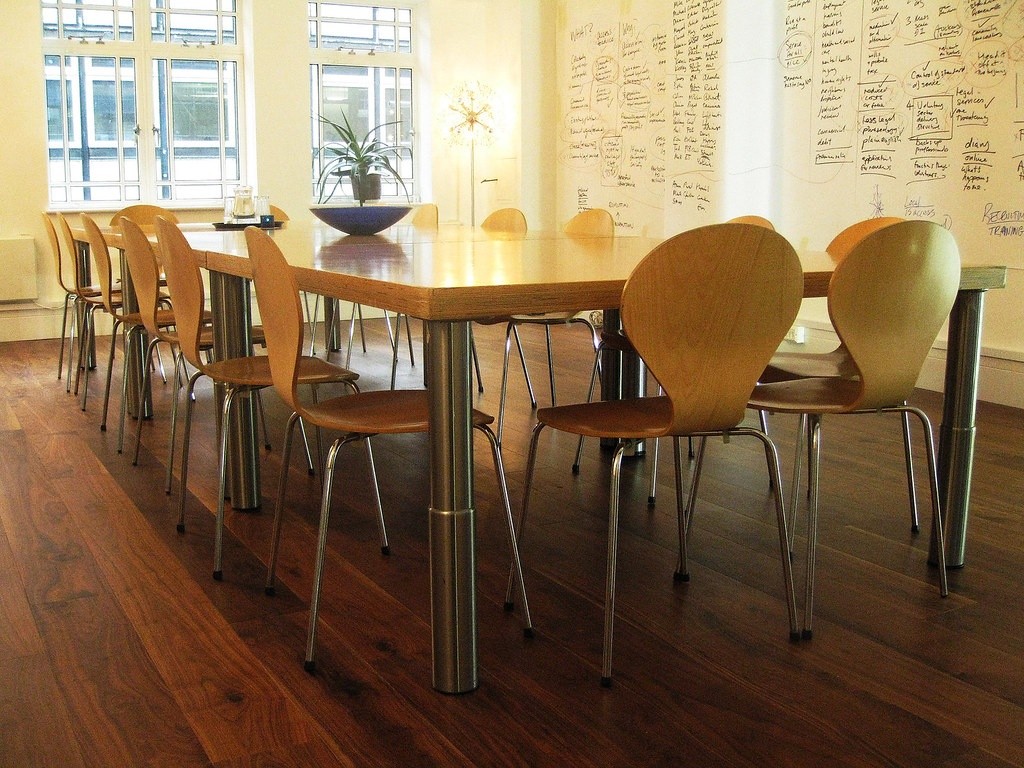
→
[310,107,413,207]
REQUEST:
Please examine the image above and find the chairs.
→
[42,207,961,682]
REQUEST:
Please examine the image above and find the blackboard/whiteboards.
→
[535,0,1024,364]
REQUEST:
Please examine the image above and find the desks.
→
[71,223,1011,701]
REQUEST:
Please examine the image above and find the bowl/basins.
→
[308,203,415,236]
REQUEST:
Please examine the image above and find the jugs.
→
[232,186,255,218]
[254,197,271,221]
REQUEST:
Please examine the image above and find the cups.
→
[260,215,274,230]
[224,197,237,224]
